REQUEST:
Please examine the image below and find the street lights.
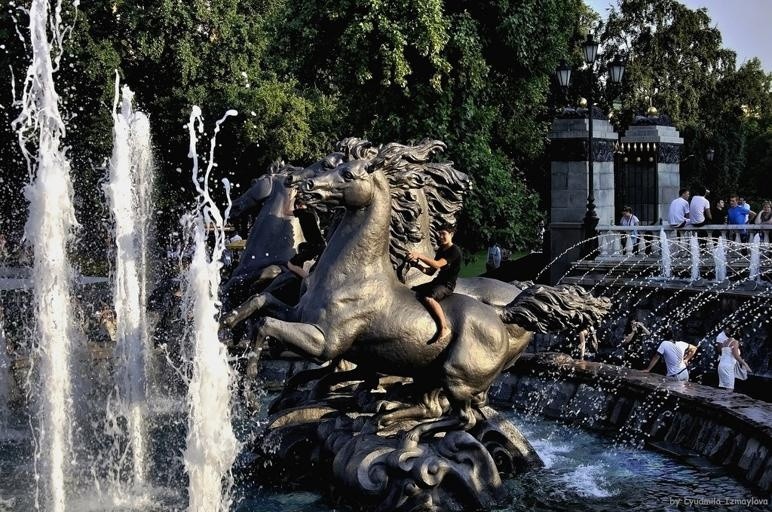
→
[555,31,627,260]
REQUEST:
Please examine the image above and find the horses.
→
[218,136,614,452]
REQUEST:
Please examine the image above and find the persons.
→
[84,227,248,354]
[714,324,729,362]
[639,326,698,381]
[668,186,771,262]
[619,205,640,256]
[406,223,463,344]
[578,324,599,361]
[715,326,752,392]
[485,238,501,273]
[0,233,36,269]
[282,186,327,280]
[622,314,651,369]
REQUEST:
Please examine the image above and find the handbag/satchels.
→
[734,361,747,381]
